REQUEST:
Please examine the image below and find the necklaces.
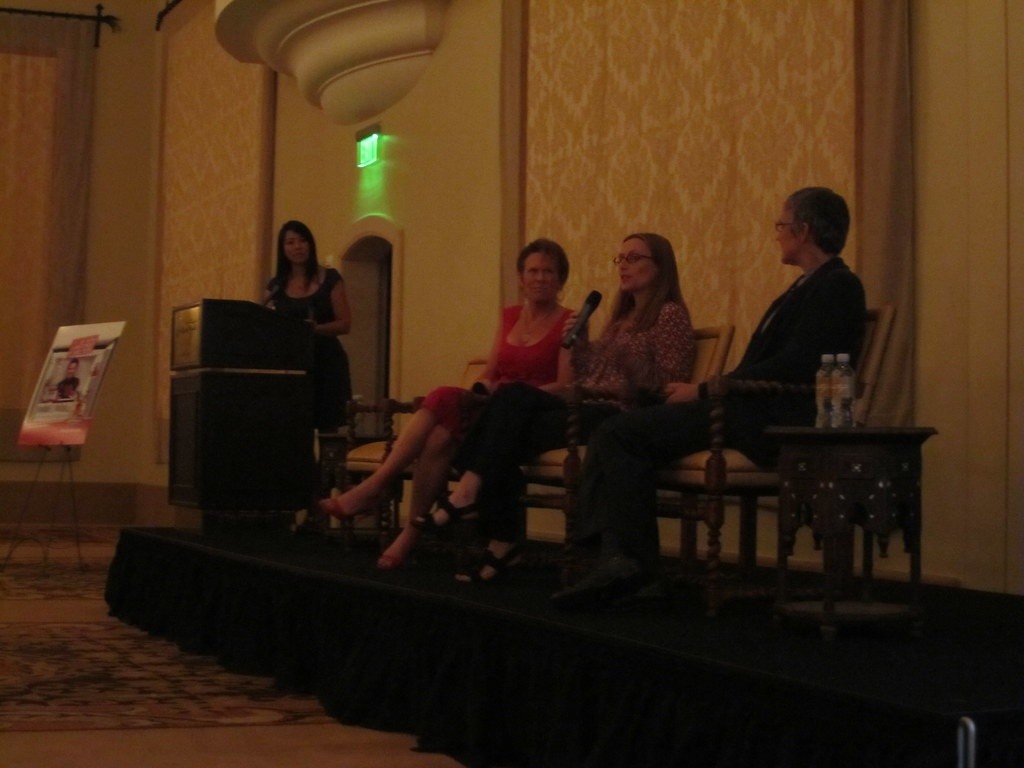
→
[520,310,552,344]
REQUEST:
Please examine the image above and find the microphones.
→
[264,282,284,308]
[561,290,602,350]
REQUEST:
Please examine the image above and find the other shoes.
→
[372,520,434,571]
[318,480,390,521]
[287,507,317,534]
[321,481,354,543]
[546,552,648,607]
[604,565,685,614]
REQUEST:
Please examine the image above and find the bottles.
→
[815,354,834,427]
[830,354,856,427]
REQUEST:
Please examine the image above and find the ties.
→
[776,284,797,310]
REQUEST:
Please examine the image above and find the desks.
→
[764,425,935,639]
[315,431,398,508]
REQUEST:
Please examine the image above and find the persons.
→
[316,240,589,570]
[555,188,866,611]
[47,358,80,399]
[408,233,699,586]
[262,221,351,535]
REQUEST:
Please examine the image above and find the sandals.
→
[410,496,486,532]
[454,545,527,587]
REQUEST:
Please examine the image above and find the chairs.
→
[344,303,903,614]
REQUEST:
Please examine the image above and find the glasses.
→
[775,218,813,233]
[613,253,652,267]
